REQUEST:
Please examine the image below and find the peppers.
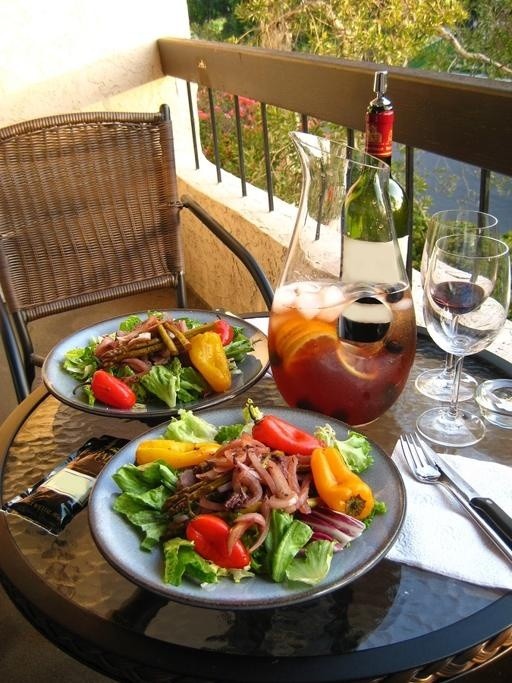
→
[249,403,323,467]
[73,370,136,409]
[183,493,250,569]
[310,447,374,521]
[188,331,232,394]
[214,320,234,346]
[134,439,221,469]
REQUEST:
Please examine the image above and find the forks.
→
[399,433,512,564]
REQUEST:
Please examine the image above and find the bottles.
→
[338,70,412,343]
[268,129,417,428]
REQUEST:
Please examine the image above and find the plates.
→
[87,405,408,611]
[41,309,270,419]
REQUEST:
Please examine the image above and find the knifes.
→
[415,432,512,559]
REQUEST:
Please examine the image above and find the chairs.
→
[1,103,276,404]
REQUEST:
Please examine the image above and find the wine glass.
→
[414,209,498,403]
[416,234,511,447]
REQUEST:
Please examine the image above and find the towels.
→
[382,438,512,591]
